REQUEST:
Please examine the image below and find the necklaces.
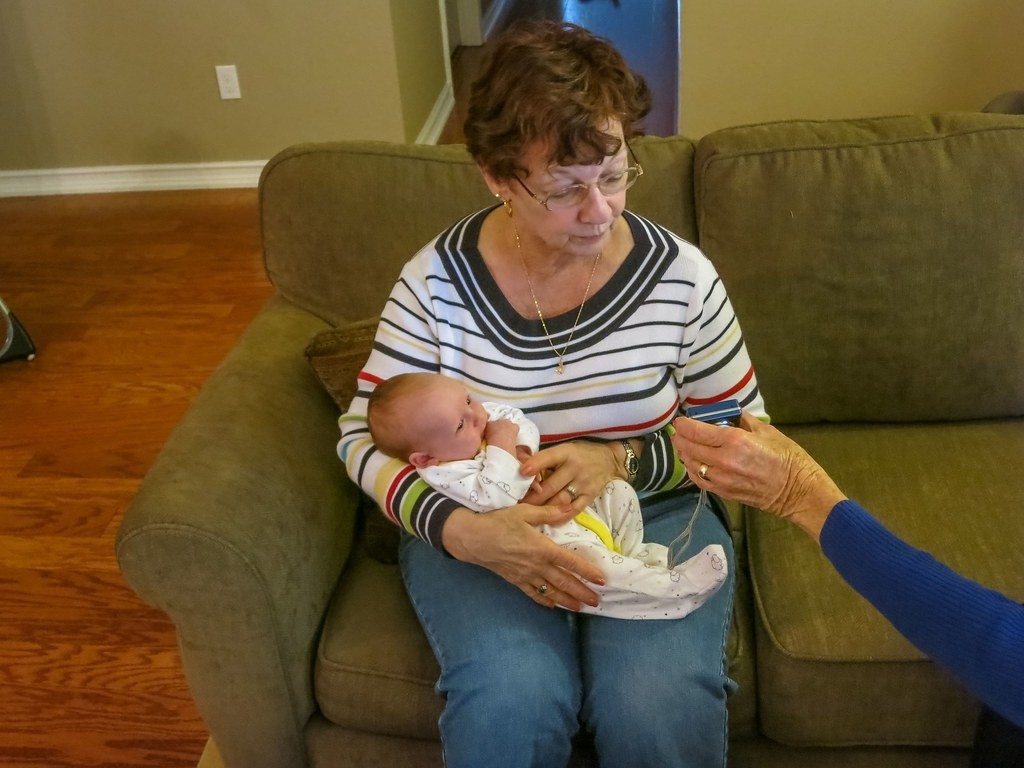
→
[514,221,600,375]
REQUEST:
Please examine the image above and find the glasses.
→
[512,137,644,212]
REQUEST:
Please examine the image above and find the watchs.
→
[620,439,640,484]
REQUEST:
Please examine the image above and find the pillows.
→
[305,317,400,564]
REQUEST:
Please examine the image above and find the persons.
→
[367,372,729,621]
[334,28,774,768]
[665,406,1024,768]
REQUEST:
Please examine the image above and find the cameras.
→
[686,399,743,428]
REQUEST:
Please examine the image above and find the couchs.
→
[114,92,1023,768]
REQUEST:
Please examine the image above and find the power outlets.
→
[216,64,241,100]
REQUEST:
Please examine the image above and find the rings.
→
[564,485,577,500]
[537,583,548,594]
[698,464,708,479]
